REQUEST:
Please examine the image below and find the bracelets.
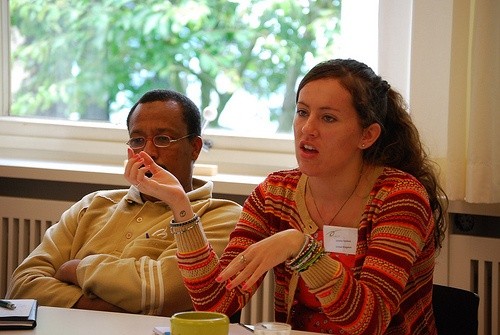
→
[286,232,327,272]
[169,217,199,234]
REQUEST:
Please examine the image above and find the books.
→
[0,300,37,329]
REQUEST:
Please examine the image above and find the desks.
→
[0,306,333,335]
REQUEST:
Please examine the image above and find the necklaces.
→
[308,156,361,226]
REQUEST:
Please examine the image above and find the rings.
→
[240,256,248,267]
[135,184,140,187]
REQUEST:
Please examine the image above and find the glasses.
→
[126,133,192,150]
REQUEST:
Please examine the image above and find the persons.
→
[5,90,237,318]
[123,59,452,335]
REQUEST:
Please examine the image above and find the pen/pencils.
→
[145,232,150,238]
[2,300,17,310]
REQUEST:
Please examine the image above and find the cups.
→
[254,321,291,335]
[170,311,230,335]
